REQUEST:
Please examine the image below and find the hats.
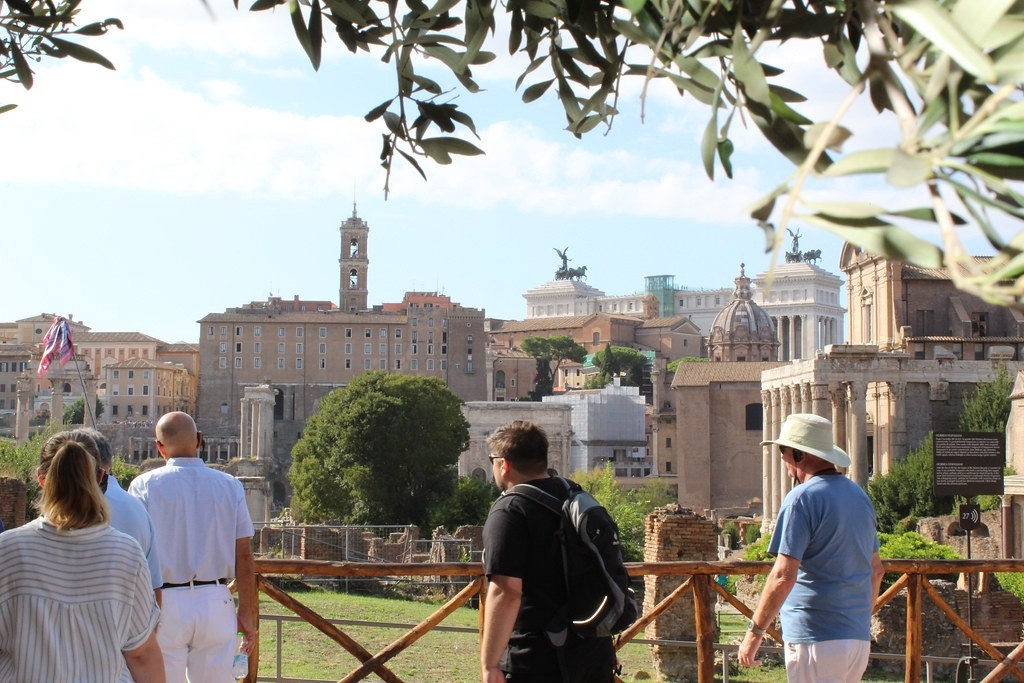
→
[760,413,851,467]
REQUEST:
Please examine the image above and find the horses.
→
[569,265,588,282]
[804,249,823,264]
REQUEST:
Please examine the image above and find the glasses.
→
[489,455,504,464]
[779,445,792,454]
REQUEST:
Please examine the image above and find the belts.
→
[161,578,226,588]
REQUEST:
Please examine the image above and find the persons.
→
[736,413,884,683]
[128,411,258,683]
[477,421,618,683]
[0,428,162,683]
[716,574,727,606]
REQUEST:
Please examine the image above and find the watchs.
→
[748,621,767,634]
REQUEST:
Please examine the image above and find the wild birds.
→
[553,247,569,259]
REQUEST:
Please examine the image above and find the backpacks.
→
[483,476,638,637]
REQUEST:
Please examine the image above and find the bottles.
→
[232,633,248,679]
